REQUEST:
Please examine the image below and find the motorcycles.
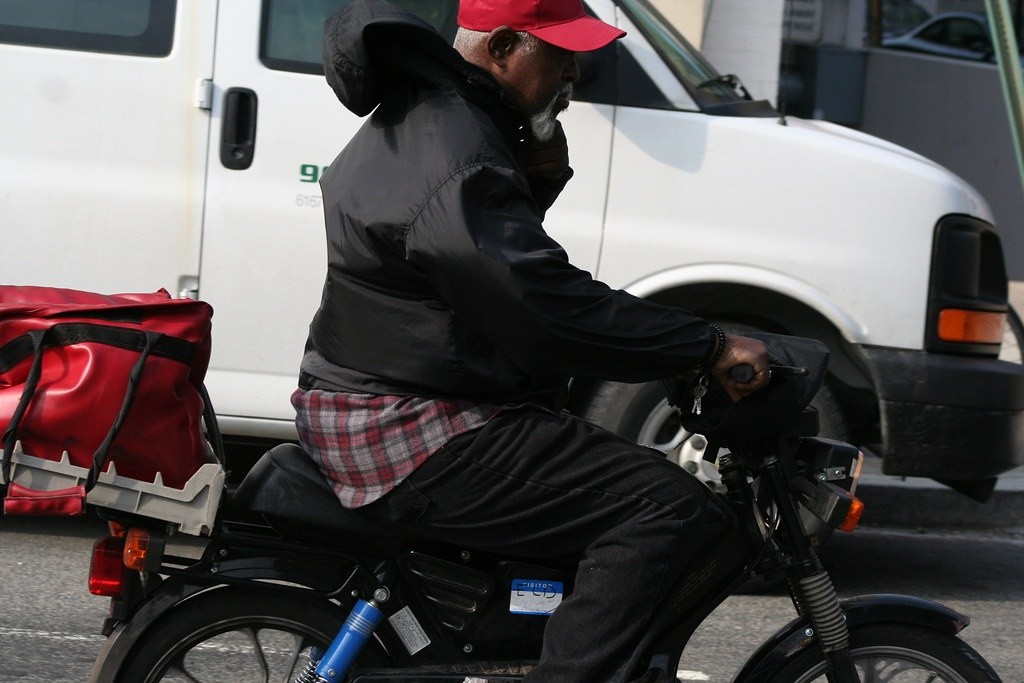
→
[94,356,1005,681]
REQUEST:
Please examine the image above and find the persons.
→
[292,0,772,683]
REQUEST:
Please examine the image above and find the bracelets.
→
[692,324,726,376]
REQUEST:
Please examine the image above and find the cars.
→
[882,13,998,66]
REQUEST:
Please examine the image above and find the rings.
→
[768,370,772,378]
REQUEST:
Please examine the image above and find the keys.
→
[692,385,707,416]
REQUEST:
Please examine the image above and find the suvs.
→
[0,0,1024,502]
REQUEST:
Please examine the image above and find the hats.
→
[458,0,628,53]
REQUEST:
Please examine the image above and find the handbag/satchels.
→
[1,284,213,518]
